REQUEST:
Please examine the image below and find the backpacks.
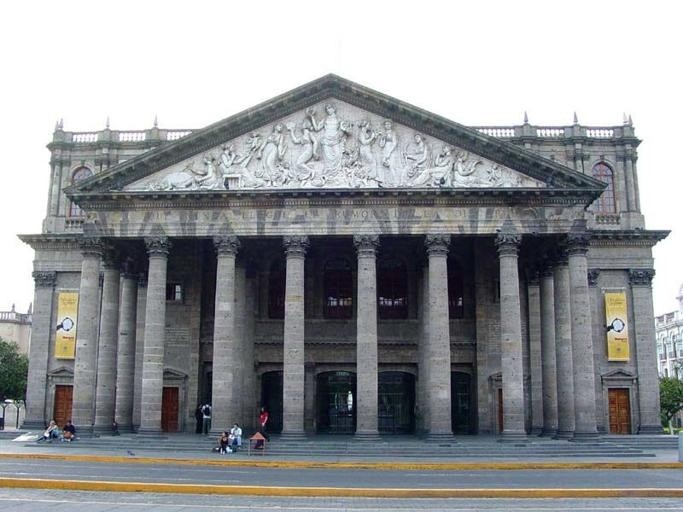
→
[203,405,211,415]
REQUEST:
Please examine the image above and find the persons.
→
[195,401,203,433]
[200,400,212,435]
[230,424,242,451]
[231,438,238,452]
[36,420,59,443]
[62,419,76,441]
[217,431,229,455]
[259,406,270,442]
